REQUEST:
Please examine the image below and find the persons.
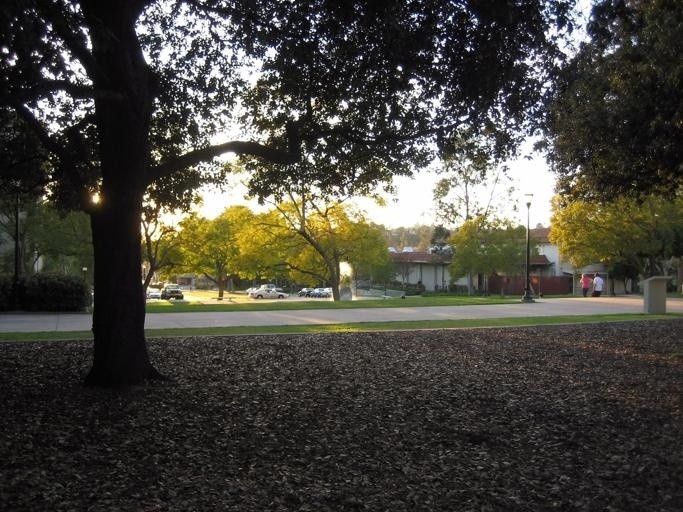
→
[589,273,604,296]
[579,272,593,296]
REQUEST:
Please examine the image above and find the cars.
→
[402,246,413,253]
[298,288,333,297]
[147,284,184,300]
[246,284,289,299]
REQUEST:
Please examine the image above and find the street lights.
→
[520,193,535,303]
[12,176,22,276]
